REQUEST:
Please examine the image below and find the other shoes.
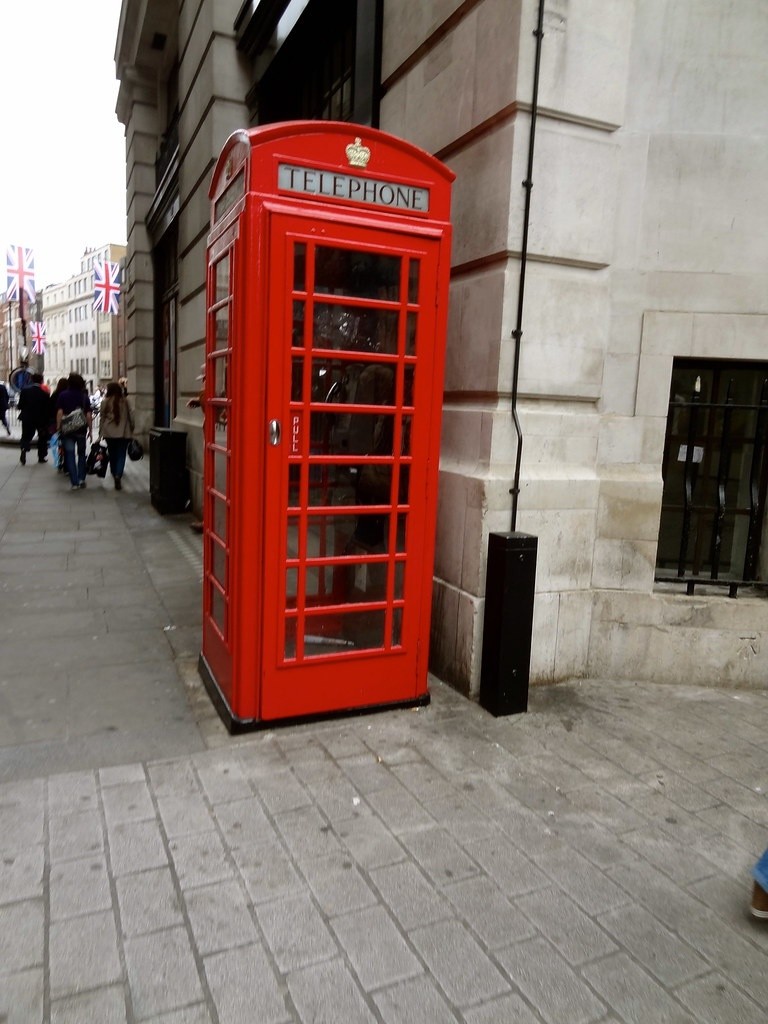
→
[72,484,79,489]
[20,447,27,465]
[38,457,49,463]
[114,473,122,490]
[79,479,87,489]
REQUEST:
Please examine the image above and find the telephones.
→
[325,363,378,451]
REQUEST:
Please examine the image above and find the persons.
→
[87,376,129,427]
[96,382,135,490]
[186,362,208,534]
[46,377,68,473]
[15,374,51,465]
[0,383,11,437]
[56,372,92,490]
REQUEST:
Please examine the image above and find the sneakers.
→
[750,871,768,918]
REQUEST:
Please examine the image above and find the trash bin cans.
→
[146,424,192,516]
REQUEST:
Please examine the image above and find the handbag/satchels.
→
[86,442,110,478]
[61,407,88,436]
[128,438,144,461]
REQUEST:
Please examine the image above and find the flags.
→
[93,259,121,315]
[31,320,46,354]
[6,244,36,302]
